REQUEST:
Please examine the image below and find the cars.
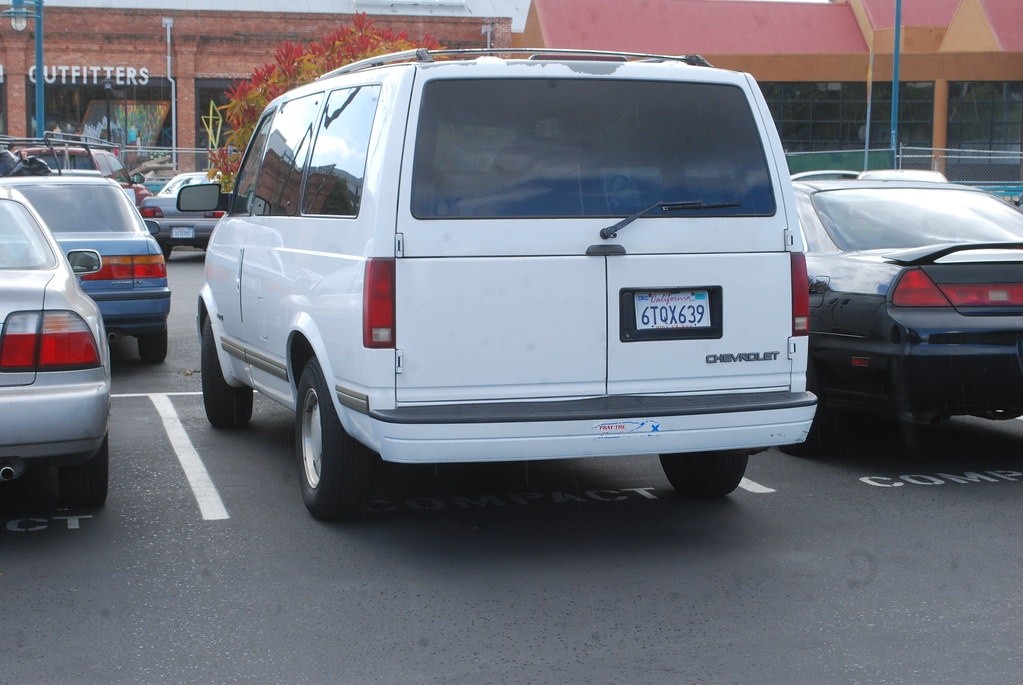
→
[779,170,1023,464]
[138,172,234,262]
[0,176,172,366]
[0,185,110,512]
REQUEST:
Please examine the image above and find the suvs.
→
[0,131,153,213]
[177,48,819,526]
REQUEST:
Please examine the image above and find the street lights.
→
[0,1,46,138]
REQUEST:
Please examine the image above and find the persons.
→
[9,143,16,152]
